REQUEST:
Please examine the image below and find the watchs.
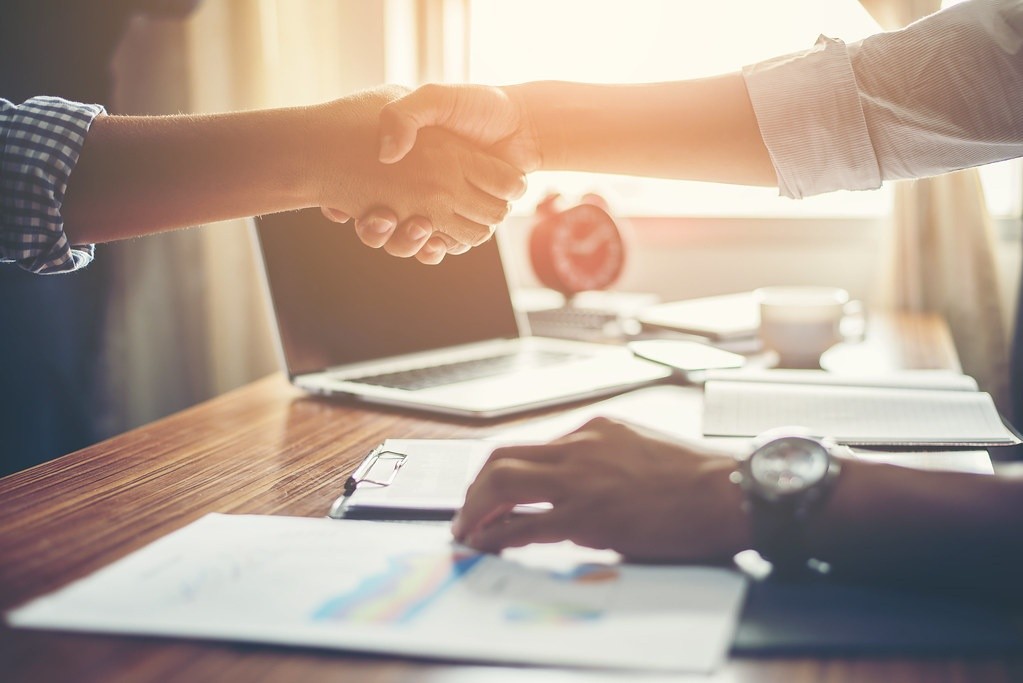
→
[732,428,850,573]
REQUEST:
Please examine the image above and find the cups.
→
[752,285,868,369]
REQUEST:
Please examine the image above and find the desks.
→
[0,304,1022,683]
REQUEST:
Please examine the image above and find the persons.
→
[3,86,528,275]
[322,0,1023,265]
[451,415,1023,581]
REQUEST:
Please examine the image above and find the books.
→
[702,366,1020,450]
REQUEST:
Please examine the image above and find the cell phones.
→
[627,339,743,371]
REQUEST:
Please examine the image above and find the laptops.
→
[253,207,673,420]
